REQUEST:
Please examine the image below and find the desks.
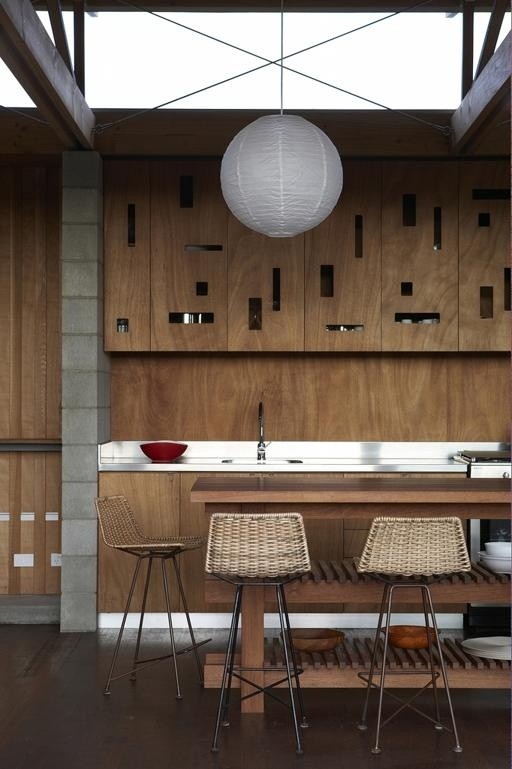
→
[190,477,512,713]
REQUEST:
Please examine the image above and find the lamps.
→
[219,0,343,238]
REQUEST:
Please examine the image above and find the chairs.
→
[204,512,310,756]
[352,515,470,755]
[94,496,208,699]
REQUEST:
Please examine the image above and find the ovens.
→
[465,516,512,665]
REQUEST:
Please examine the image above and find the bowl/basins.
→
[377,625,443,650]
[139,443,187,463]
[477,541,511,575]
[279,627,345,653]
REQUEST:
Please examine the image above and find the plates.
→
[454,638,512,667]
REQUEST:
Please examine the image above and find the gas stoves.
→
[459,441,512,483]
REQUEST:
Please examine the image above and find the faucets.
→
[258,402,264,442]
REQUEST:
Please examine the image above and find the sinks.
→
[223,459,303,465]
